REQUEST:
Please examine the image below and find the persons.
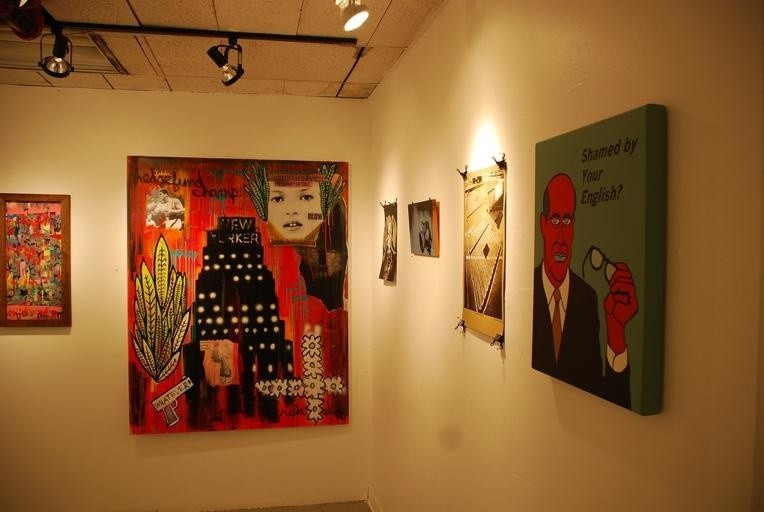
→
[383,215,397,283]
[532,173,638,411]
[146,189,185,230]
[268,181,323,240]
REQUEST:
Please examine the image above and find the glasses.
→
[582,245,617,282]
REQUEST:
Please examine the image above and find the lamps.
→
[38,29,75,79]
[0,1,44,42]
[207,38,245,87]
[335,1,369,33]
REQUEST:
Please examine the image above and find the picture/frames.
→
[0,192,73,328]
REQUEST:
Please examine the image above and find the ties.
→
[552,289,562,364]
